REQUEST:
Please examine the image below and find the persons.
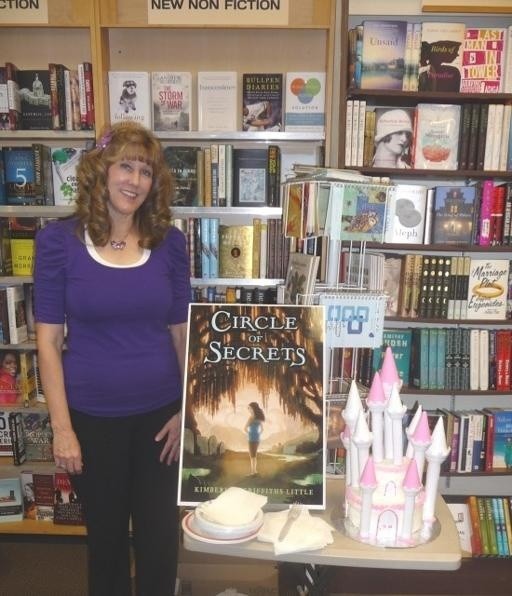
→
[31,123,190,595]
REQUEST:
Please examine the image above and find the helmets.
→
[182,511,265,544]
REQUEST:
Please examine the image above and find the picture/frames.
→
[183,478,462,595]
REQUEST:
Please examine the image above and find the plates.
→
[95,1,335,538]
[279,1,512,595]
[0,2,100,536]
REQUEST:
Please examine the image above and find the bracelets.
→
[193,500,263,539]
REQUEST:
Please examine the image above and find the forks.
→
[278,500,303,542]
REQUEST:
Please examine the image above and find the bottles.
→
[374,109,414,144]
